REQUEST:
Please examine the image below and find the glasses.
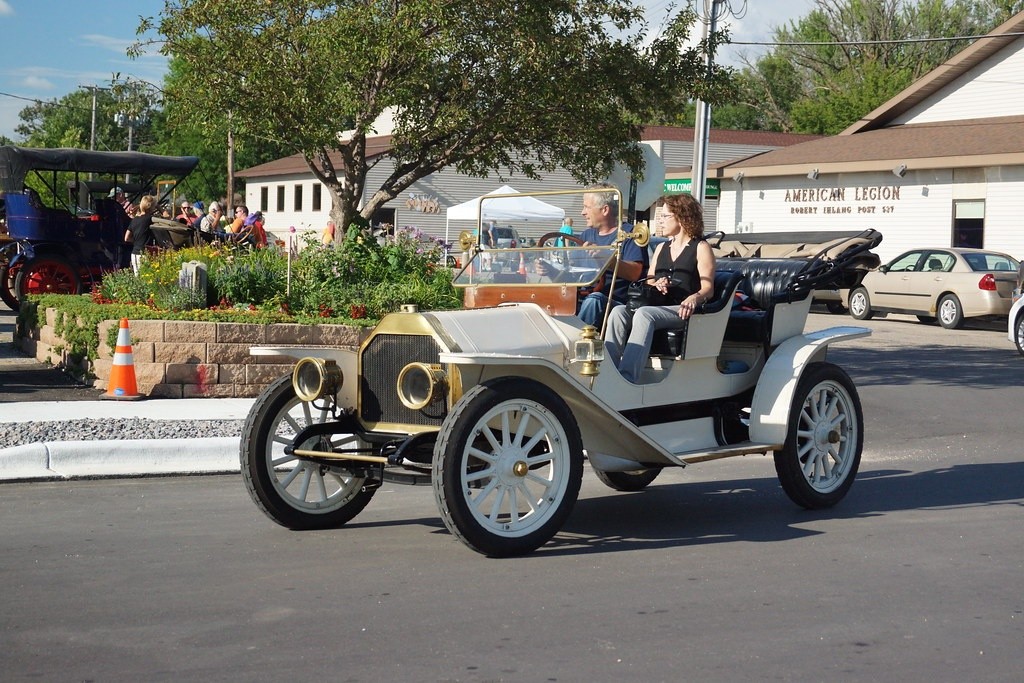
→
[234,210,242,214]
[182,207,190,209]
[659,213,673,218]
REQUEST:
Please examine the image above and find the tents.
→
[444,184,566,269]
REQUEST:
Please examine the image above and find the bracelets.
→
[694,292,707,303]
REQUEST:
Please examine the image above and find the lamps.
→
[892,164,907,178]
[807,168,820,181]
[733,171,744,183]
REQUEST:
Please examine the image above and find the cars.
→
[848,246,1020,329]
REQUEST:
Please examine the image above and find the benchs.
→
[4,186,80,242]
[651,270,744,360]
[714,259,808,341]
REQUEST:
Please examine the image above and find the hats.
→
[255,211,264,216]
[107,186,123,197]
[194,201,204,209]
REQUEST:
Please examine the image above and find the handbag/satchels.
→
[626,275,683,313]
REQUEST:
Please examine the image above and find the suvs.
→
[493,224,527,272]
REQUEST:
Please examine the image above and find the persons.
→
[107,187,269,278]
[477,219,498,272]
[321,220,335,253]
[535,182,716,384]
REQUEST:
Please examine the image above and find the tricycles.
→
[0,144,262,313]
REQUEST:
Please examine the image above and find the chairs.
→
[929,259,943,271]
[94,198,134,247]
[994,262,1009,271]
[151,217,197,250]
